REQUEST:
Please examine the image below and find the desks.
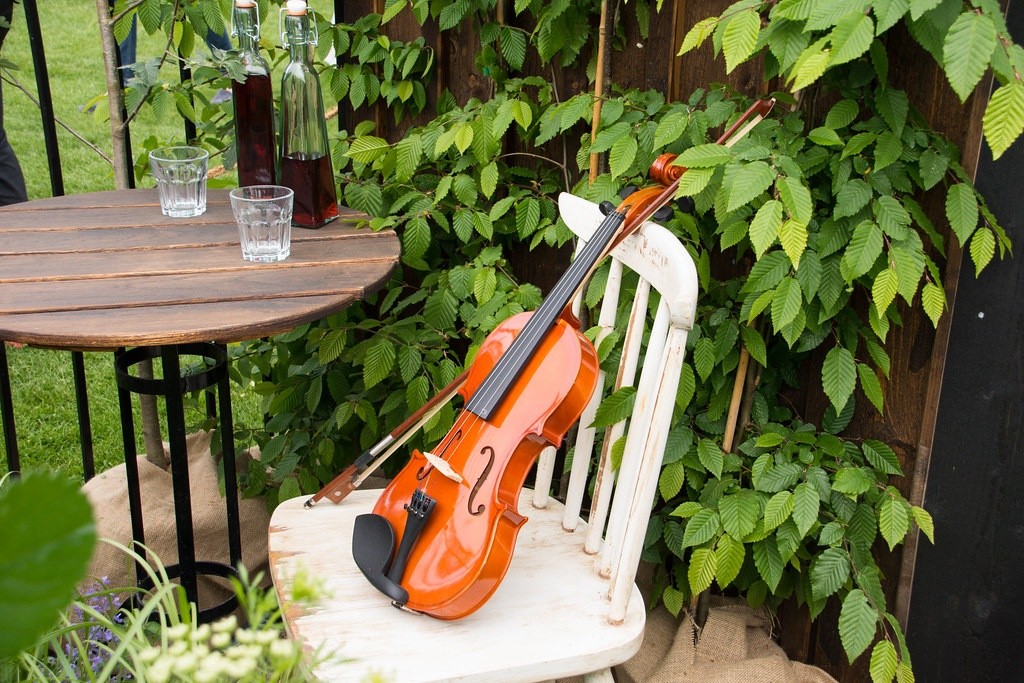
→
[0,187,405,665]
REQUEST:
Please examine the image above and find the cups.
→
[229,185,295,262]
[148,146,209,218]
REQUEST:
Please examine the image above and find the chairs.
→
[268,190,698,683]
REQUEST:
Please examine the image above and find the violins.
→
[351,153,689,622]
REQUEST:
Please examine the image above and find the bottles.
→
[231,0,277,187]
[278,0,340,230]
